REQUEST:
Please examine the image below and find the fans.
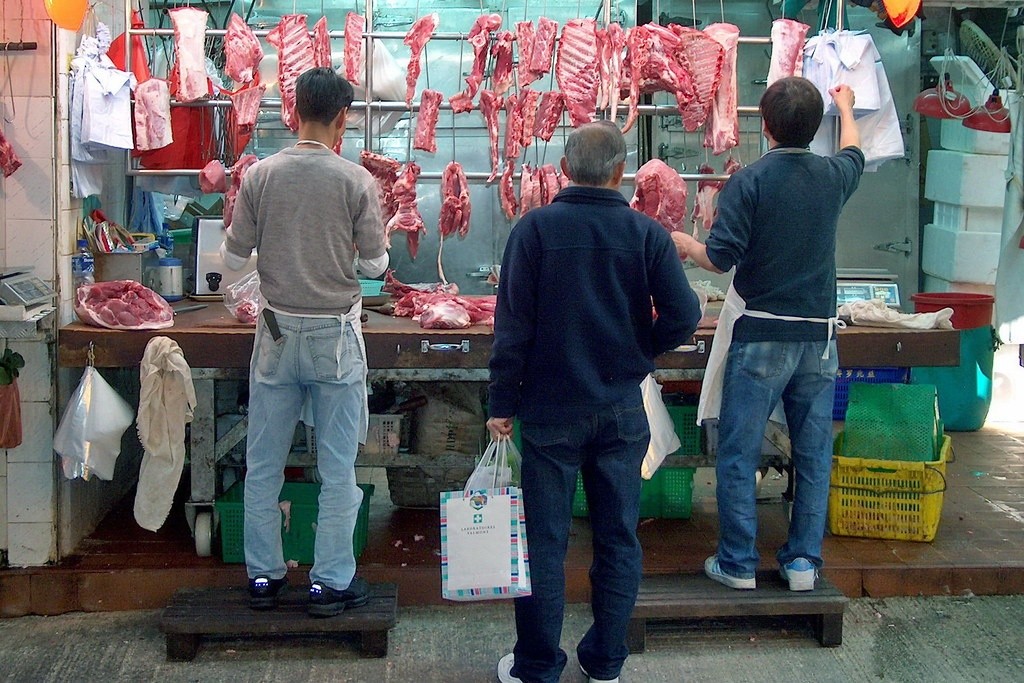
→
[959,20,1019,90]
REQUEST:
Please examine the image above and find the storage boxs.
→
[832,366,911,418]
[216,413,314,468]
[922,277,996,326]
[827,430,952,542]
[307,411,408,462]
[840,380,944,508]
[923,150,1007,209]
[939,116,1011,156]
[214,482,375,564]
[920,224,1000,285]
[561,404,702,519]
[934,200,1004,233]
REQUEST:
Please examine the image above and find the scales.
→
[834,267,901,308]
[0,265,58,309]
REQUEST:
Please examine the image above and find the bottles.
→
[71,239,95,291]
[158,257,183,301]
[157,223,175,258]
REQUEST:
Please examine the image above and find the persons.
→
[671,77,865,591]
[485,120,703,683]
[218,67,390,617]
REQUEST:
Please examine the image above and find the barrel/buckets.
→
[911,293,994,329]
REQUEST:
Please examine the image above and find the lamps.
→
[915,2,972,119]
[962,8,1020,132]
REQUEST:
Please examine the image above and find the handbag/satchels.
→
[439,430,533,601]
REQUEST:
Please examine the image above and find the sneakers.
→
[249,574,289,610]
[497,654,522,683]
[703,553,756,588]
[307,574,373,616]
[580,662,620,683]
[777,556,818,591]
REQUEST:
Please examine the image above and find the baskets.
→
[829,429,951,542]
[305,414,402,457]
[212,479,376,563]
[840,382,945,470]
[573,466,695,518]
[666,407,701,457]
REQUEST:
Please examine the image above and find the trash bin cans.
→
[907,292,1004,432]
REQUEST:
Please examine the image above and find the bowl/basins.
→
[361,292,392,306]
